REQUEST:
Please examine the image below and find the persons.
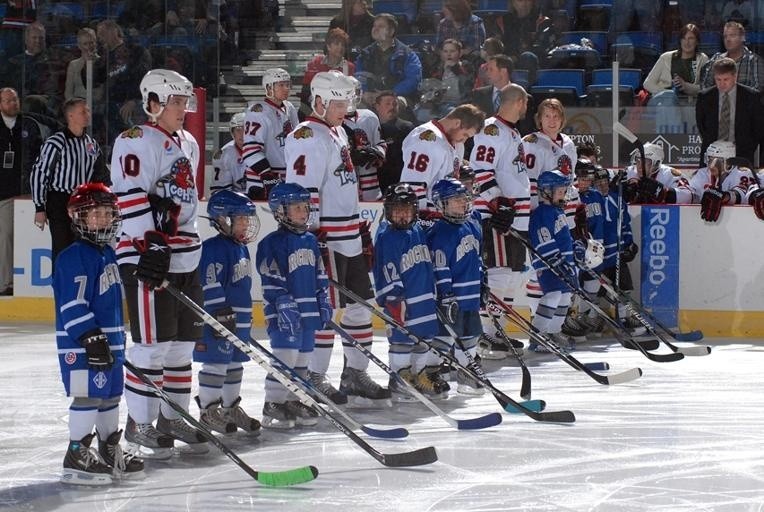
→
[464,82,533,359]
[609,143,693,204]
[354,13,421,110]
[241,66,300,203]
[689,135,756,223]
[463,54,537,159]
[52,181,145,485]
[642,22,710,107]
[525,170,576,356]
[431,37,475,115]
[296,28,356,124]
[399,102,486,218]
[372,184,450,400]
[374,93,415,192]
[284,70,393,410]
[596,167,648,337]
[746,167,764,220]
[343,76,387,204]
[330,0,376,59]
[699,20,764,93]
[212,113,250,195]
[2,0,277,93]
[31,96,112,286]
[572,160,607,339]
[576,140,620,196]
[195,190,262,445]
[473,37,504,90]
[495,0,561,64]
[257,184,333,431]
[520,99,588,344]
[0,86,40,296]
[696,57,764,169]
[427,179,489,395]
[110,68,212,460]
[436,0,486,70]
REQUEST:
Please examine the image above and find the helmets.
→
[537,171,571,207]
[309,71,354,118]
[261,68,291,99]
[581,237,604,272]
[631,143,663,175]
[268,183,311,235]
[576,163,595,192]
[593,170,611,197]
[140,69,194,118]
[208,191,260,245]
[68,183,124,244]
[347,76,361,107]
[432,178,474,225]
[704,141,737,173]
[229,114,247,138]
[384,183,419,230]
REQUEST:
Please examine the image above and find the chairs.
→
[345,0,698,108]
[35,0,220,62]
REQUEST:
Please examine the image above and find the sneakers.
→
[596,319,624,334]
[263,402,298,420]
[561,314,584,336]
[575,315,600,333]
[479,300,503,318]
[387,371,422,395]
[286,397,319,418]
[220,397,263,431]
[123,415,174,447]
[96,431,144,471]
[616,314,645,328]
[438,364,448,372]
[200,400,238,435]
[156,410,209,443]
[458,366,484,389]
[306,374,349,404]
[415,368,450,393]
[477,333,508,352]
[63,435,114,474]
[550,332,574,347]
[488,330,525,350]
[529,334,559,346]
[341,367,392,399]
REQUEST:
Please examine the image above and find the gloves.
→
[627,175,641,192]
[574,241,587,262]
[355,144,384,169]
[700,185,724,223]
[275,294,302,340]
[136,231,171,292]
[480,280,491,307]
[260,172,279,201]
[547,253,575,283]
[360,224,375,272]
[384,297,406,327]
[575,207,588,240]
[749,187,764,218]
[491,199,514,232]
[81,332,112,371]
[316,291,333,328]
[318,234,331,268]
[215,308,236,340]
[437,292,459,326]
[637,177,667,204]
[622,244,639,262]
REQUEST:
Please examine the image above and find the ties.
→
[718,89,732,143]
[494,88,504,114]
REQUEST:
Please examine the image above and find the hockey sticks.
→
[575,283,660,351]
[326,320,503,431]
[486,300,643,387]
[111,350,320,488]
[726,156,762,188]
[483,301,533,401]
[330,279,576,426]
[247,335,410,441]
[513,309,610,372]
[578,262,712,356]
[612,121,647,177]
[435,305,546,414]
[485,202,685,362]
[612,285,703,342]
[614,184,647,338]
[160,280,439,469]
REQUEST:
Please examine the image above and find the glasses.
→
[435,89,448,99]
[478,44,485,49]
[382,100,397,106]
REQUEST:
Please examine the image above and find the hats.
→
[419,78,451,103]
